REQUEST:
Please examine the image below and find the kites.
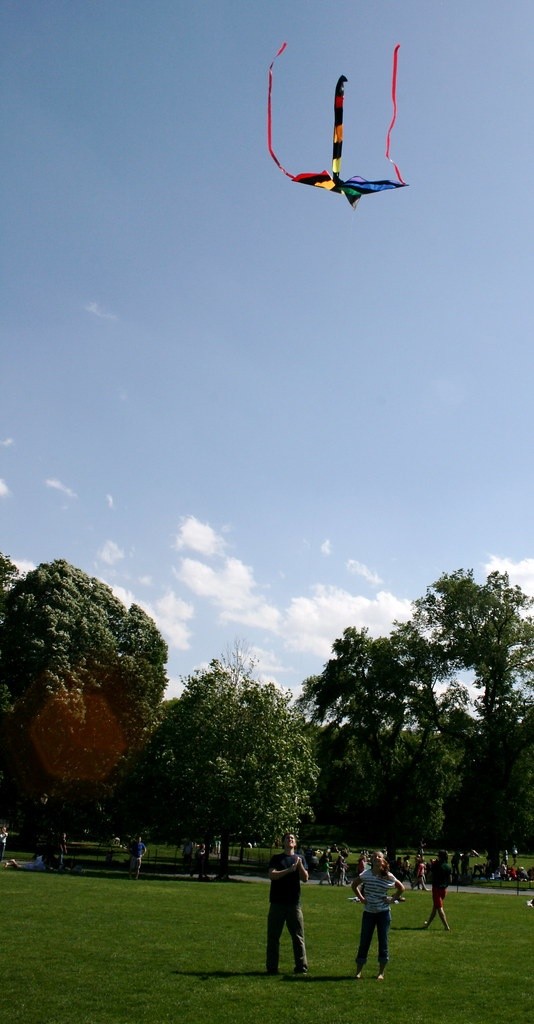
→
[267,42,411,209]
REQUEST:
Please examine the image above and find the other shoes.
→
[356,973,361,978]
[300,970,307,975]
[377,974,384,980]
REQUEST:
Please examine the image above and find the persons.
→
[58,833,67,873]
[183,840,207,881]
[4,855,47,871]
[424,850,453,932]
[0,826,7,862]
[303,838,534,890]
[129,836,147,879]
[352,852,405,981]
[266,833,309,975]
